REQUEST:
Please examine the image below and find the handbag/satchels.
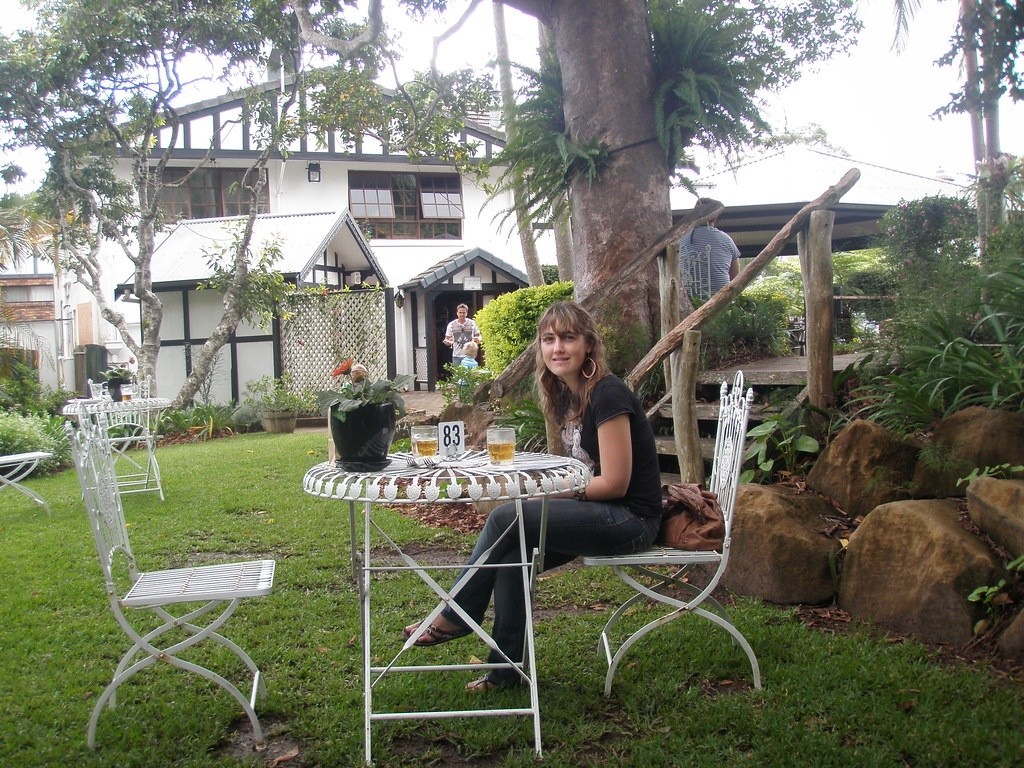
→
[655,481,725,551]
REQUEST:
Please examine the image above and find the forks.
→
[406,457,424,468]
[422,457,444,468]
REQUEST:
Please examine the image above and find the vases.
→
[330,402,397,461]
[107,378,131,401]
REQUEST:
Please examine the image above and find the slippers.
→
[465,673,519,699]
[401,617,473,646]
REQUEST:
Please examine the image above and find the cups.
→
[410,424,437,458]
[487,427,515,468]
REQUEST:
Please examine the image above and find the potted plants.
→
[244,371,312,434]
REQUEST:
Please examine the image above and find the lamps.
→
[394,288,406,309]
[305,161,321,183]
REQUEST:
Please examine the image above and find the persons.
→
[678,217,741,298]
[443,303,480,362]
[401,301,660,695]
[458,342,478,386]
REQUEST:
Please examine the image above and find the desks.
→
[62,398,184,501]
[303,450,591,768]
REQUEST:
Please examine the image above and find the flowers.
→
[311,358,417,423]
[98,357,135,379]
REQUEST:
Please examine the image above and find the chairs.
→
[65,400,276,753]
[582,368,763,696]
[88,375,161,483]
[0,451,53,518]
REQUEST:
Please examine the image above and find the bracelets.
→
[574,485,587,501]
